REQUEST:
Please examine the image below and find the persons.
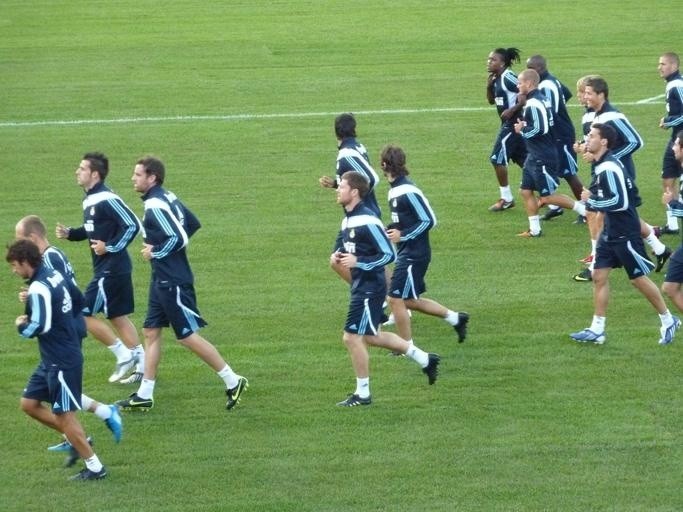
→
[112,155,250,412]
[14,214,123,450]
[6,239,107,482]
[335,171,440,405]
[318,113,388,310]
[55,152,145,383]
[487,46,683,345]
[379,145,470,356]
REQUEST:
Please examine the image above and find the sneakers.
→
[658,315,682,344]
[569,328,606,344]
[422,354,440,385]
[571,256,593,281]
[487,199,563,237]
[336,393,372,408]
[47,436,107,480]
[451,312,469,343]
[225,376,249,410]
[106,351,153,443]
[571,215,587,224]
[652,225,679,272]
